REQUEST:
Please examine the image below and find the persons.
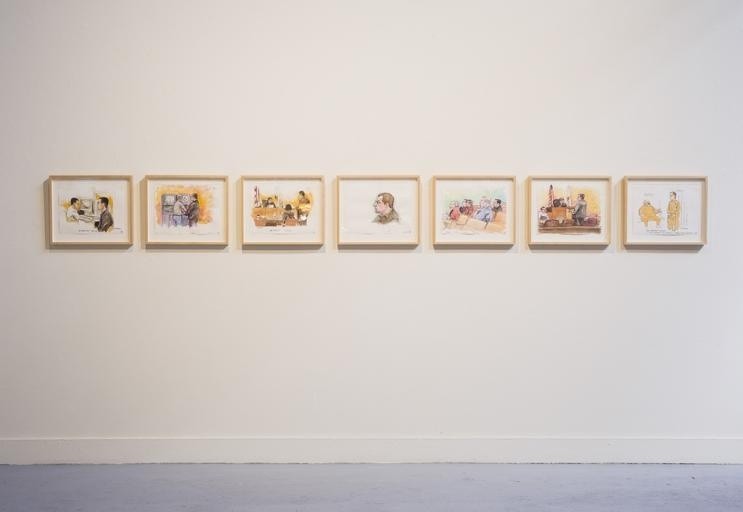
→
[447,195,502,222]
[639,200,661,228]
[185,192,199,227]
[540,188,586,225]
[173,195,186,226]
[66,197,80,222]
[283,204,294,223]
[264,197,275,208]
[298,191,309,204]
[372,193,398,224]
[96,197,113,232]
[667,191,681,231]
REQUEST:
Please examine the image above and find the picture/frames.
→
[432,175,516,246]
[336,176,420,246]
[144,174,229,246]
[526,176,611,251]
[238,176,325,245]
[48,176,133,246]
[623,177,708,247]
